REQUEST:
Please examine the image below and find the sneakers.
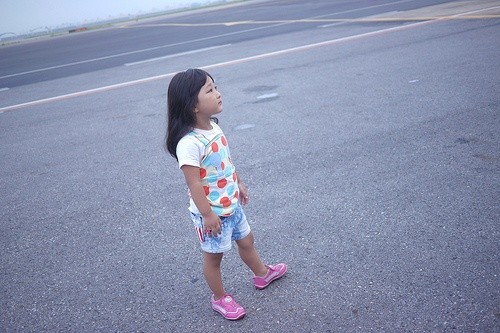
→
[210,263,288,321]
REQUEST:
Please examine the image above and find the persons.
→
[165,68,287,320]
